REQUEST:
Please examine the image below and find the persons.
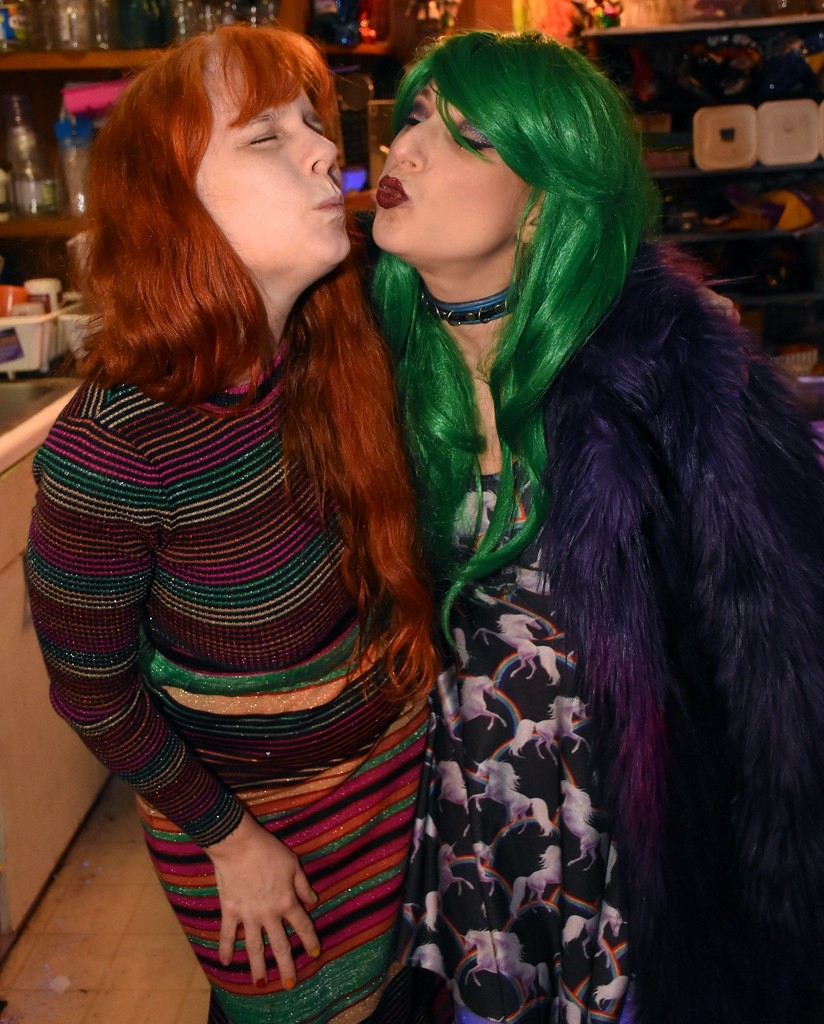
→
[21,25,432,1023]
[364,34,824,1023]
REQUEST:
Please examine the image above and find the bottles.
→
[0,88,106,222]
[2,0,288,51]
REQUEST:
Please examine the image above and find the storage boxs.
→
[0,303,97,378]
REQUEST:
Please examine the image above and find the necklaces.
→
[418,284,518,327]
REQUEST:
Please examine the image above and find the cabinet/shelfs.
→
[1,40,391,240]
[577,13,824,385]
